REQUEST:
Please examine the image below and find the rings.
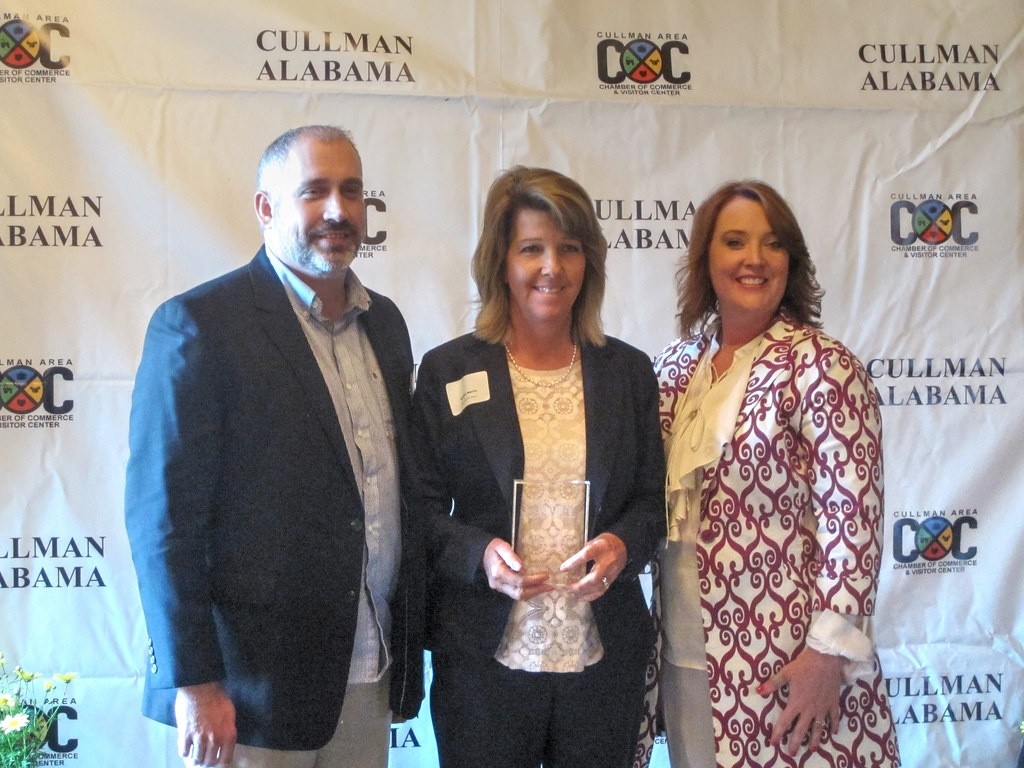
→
[815,719,825,726]
[602,577,610,590]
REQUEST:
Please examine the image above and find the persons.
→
[126,125,425,768]
[413,165,666,768]
[654,180,902,768]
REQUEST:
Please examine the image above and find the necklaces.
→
[502,338,576,387]
